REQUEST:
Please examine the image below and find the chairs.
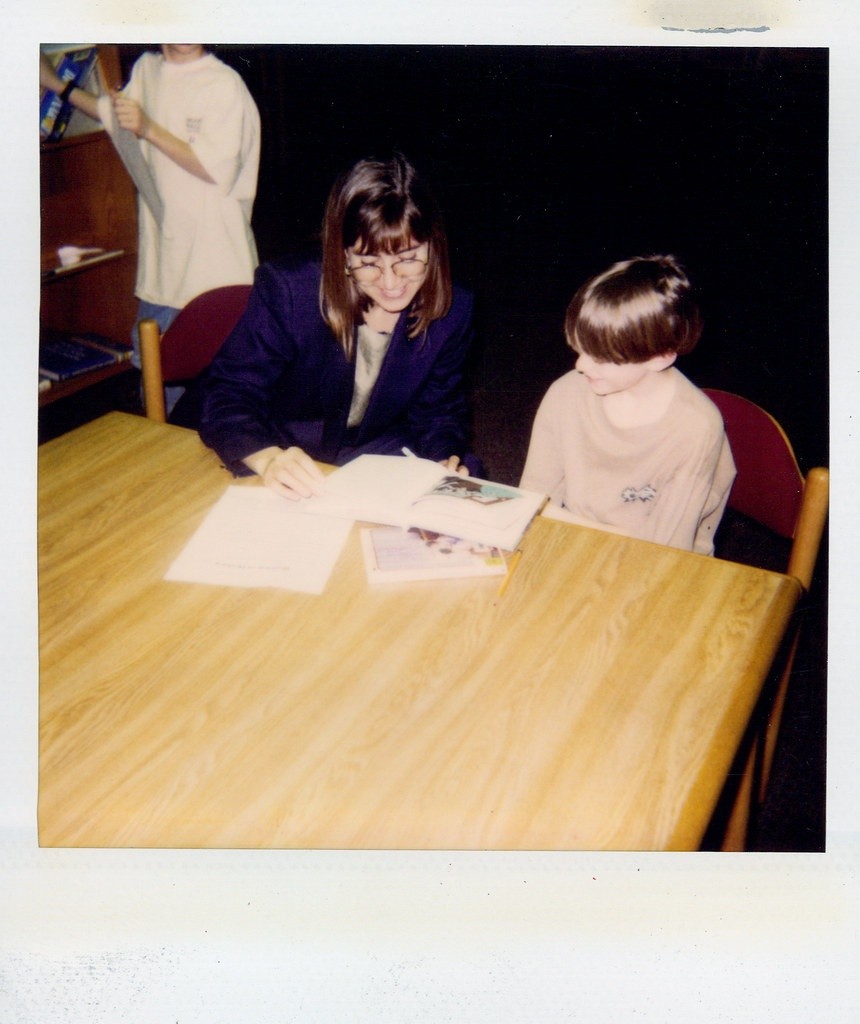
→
[702,387,828,810]
[137,283,255,424]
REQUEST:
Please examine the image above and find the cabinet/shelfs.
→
[40,43,141,446]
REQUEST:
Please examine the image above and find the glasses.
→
[342,238,431,282]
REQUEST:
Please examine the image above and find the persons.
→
[40,43,262,415]
[519,256,736,556]
[167,153,484,500]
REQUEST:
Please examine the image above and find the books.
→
[40,52,101,138]
[315,452,549,585]
[38,333,136,394]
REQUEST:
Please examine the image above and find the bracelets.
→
[60,80,77,102]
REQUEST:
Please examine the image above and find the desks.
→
[39,411,806,848]
